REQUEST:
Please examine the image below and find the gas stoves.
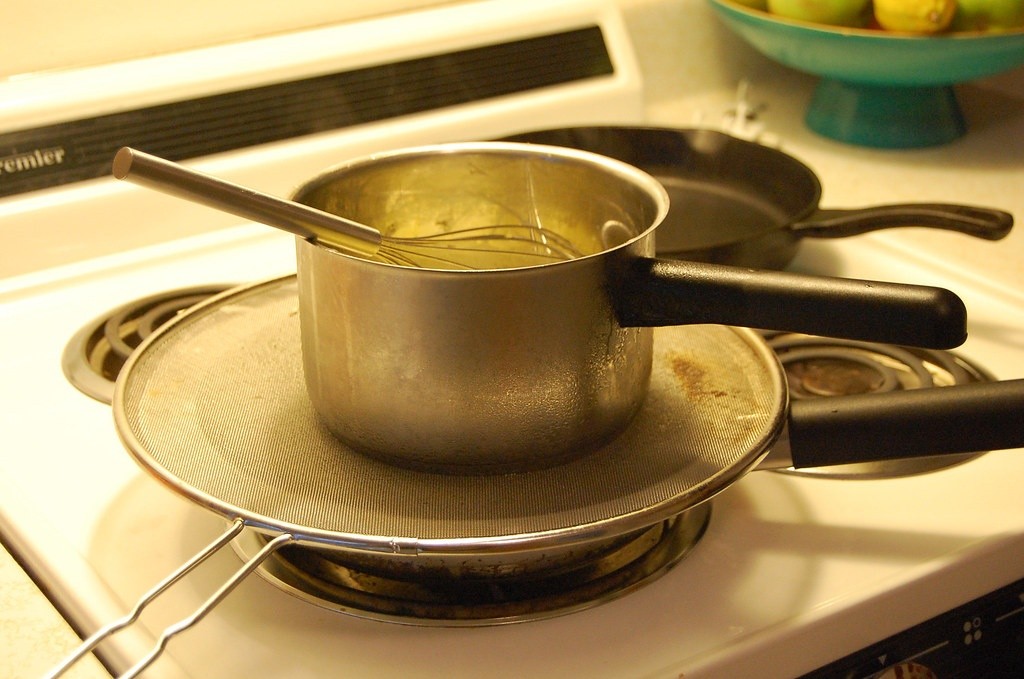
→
[0,0,1024,679]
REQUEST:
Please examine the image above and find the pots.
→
[111,271,1024,562]
[288,140,969,476]
[489,126,1015,270]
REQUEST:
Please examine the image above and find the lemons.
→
[873,0,958,33]
